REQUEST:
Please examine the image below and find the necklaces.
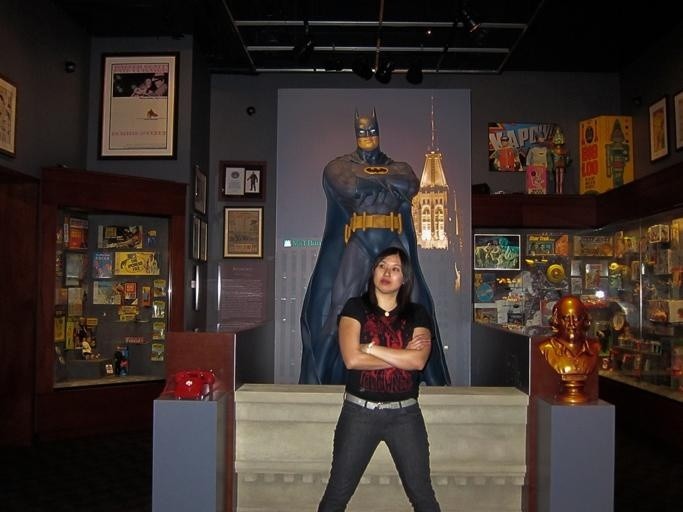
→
[376,301,397,318]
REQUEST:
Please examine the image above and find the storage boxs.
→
[578,115,634,195]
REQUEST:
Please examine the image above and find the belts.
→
[345,393,417,410]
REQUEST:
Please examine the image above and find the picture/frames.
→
[0,74,20,159]
[474,234,522,271]
[190,164,209,263]
[217,160,267,203]
[648,97,671,161]
[223,207,265,258]
[671,88,683,151]
[99,51,181,161]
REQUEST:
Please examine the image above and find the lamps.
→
[221,0,545,85]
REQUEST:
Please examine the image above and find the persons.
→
[494,135,517,171]
[297,107,451,386]
[537,295,601,376]
[315,245,442,512]
[524,130,549,195]
[142,73,167,97]
[246,172,257,191]
[548,127,571,194]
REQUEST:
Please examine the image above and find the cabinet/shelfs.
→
[35,165,187,446]
[471,161,683,406]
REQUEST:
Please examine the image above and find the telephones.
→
[173,369,215,400]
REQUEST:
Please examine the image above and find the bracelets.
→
[365,341,376,355]
